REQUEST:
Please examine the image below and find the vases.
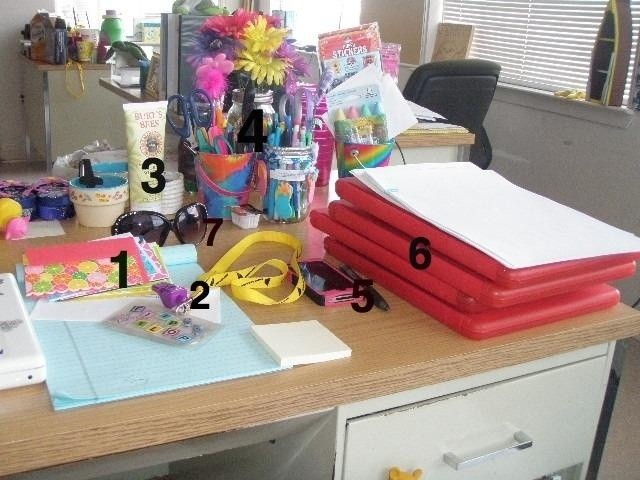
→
[226,90,278,138]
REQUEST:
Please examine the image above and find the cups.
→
[76,41,94,62]
[160,170,184,215]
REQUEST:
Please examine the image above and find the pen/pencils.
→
[196,96,235,155]
[267,114,312,148]
[340,264,390,310]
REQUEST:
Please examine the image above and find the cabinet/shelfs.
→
[97,75,475,177]
[18,53,123,174]
[0,211,640,480]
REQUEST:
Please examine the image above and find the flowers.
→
[190,9,313,93]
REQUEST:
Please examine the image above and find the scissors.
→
[167,89,213,150]
[279,88,313,129]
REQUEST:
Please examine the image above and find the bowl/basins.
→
[68,162,130,228]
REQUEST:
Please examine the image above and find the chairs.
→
[403,58,503,169]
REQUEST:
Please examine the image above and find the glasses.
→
[112,201,208,249]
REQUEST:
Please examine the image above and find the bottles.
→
[225,88,277,147]
[55,17,67,65]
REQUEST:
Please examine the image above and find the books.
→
[247,319,354,369]
[13,243,293,414]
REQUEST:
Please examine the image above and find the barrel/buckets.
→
[194,150,258,219]
[334,139,405,178]
